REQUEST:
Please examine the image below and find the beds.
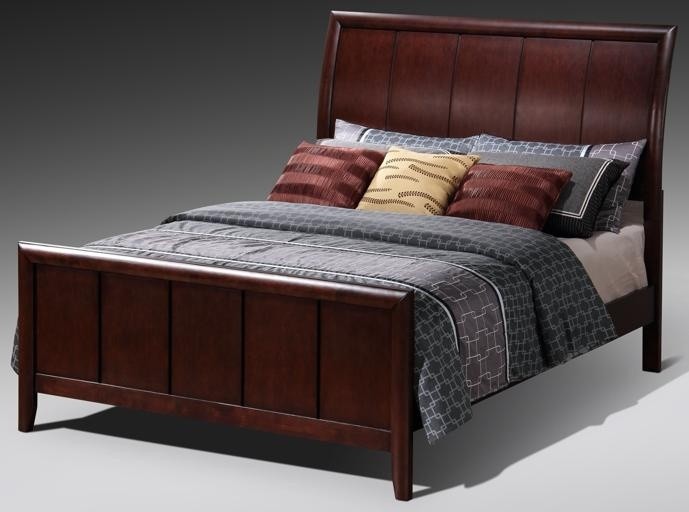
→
[17,8,680,501]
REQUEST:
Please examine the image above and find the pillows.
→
[315,119,479,154]
[472,151,632,236]
[471,133,646,234]
[266,139,386,209]
[355,145,479,216]
[445,161,572,231]
